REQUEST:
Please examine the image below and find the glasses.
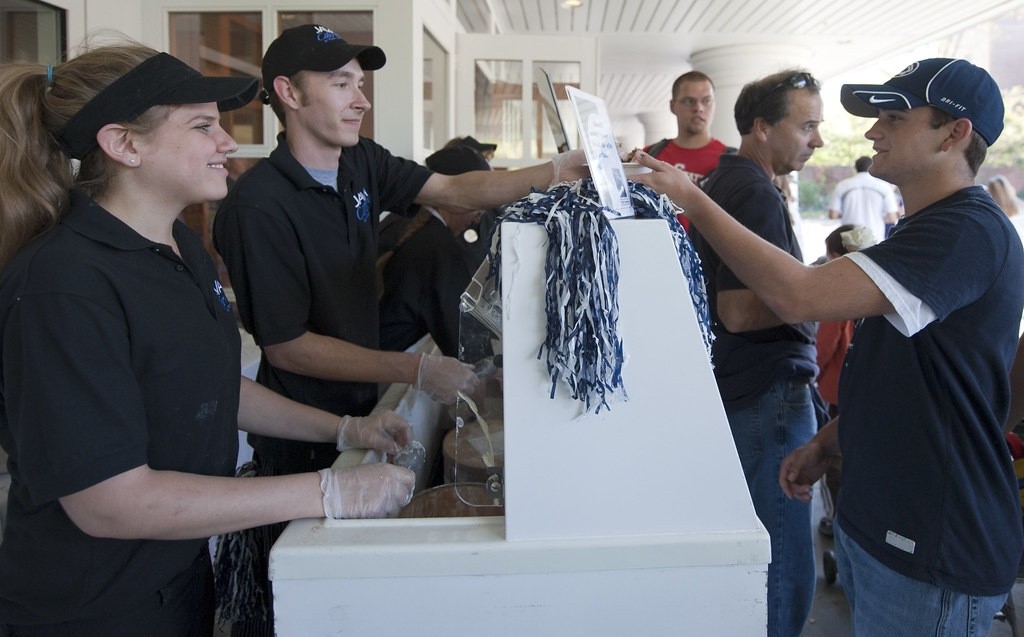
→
[752,73,820,112]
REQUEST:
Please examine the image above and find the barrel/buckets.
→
[399,418,504,518]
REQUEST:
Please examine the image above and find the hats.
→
[840,57,1004,146]
[260,24,386,104]
[460,135,497,152]
[49,52,261,162]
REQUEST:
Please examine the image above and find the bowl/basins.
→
[622,162,654,177]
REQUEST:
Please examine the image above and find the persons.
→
[375,135,498,362]
[213,26,587,563]
[618,71,729,235]
[690,69,825,637]
[803,155,1022,538]
[622,58,1024,636]
[0,43,414,637]
